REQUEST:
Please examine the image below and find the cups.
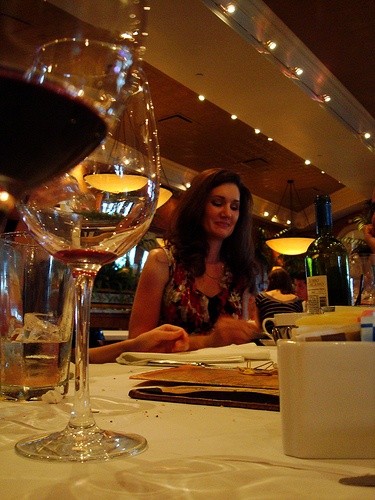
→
[261,313,362,341]
[275,340,375,459]
[348,256,374,301]
[0,231,75,402]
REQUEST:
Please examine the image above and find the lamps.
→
[84,98,148,193]
[150,142,174,209]
[264,178,316,255]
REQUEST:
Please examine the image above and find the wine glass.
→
[0,0,148,232]
[13,37,160,463]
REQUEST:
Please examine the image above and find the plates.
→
[259,338,277,346]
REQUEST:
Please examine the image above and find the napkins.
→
[116,342,271,370]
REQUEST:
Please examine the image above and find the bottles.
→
[304,194,352,305]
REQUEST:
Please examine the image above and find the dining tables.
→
[1,345,375,500]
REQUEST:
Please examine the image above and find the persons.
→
[128,168,261,351]
[0,263,190,364]
[256,190,375,347]
